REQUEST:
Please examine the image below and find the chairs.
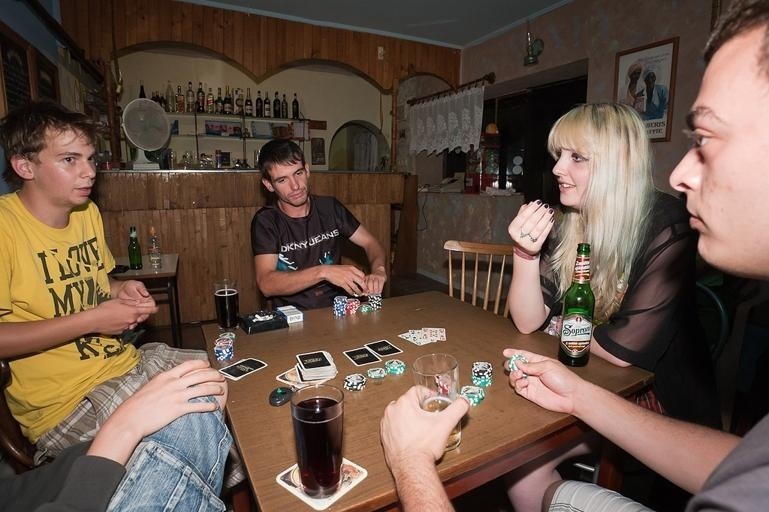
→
[443,240,513,318]
[267,255,367,312]
[564,279,728,472]
[0,359,38,470]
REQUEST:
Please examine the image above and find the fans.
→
[121,98,171,170]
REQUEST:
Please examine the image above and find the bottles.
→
[291,123,294,136]
[234,126,239,137]
[465,144,480,193]
[166,80,175,112]
[197,81,205,112]
[215,87,223,114]
[254,150,260,168]
[264,91,270,118]
[186,82,195,112]
[160,92,165,110]
[243,128,250,137]
[184,151,212,169]
[251,121,256,137]
[169,152,175,169]
[273,91,280,117]
[282,94,288,119]
[239,89,244,115]
[205,124,209,134]
[128,226,142,270]
[148,227,162,268]
[223,85,231,115]
[206,88,214,114]
[233,90,239,114]
[176,85,185,113]
[156,91,160,101]
[558,242,596,365]
[256,90,262,118]
[245,87,252,116]
[236,159,249,169]
[139,80,145,98]
[215,150,222,168]
[288,124,292,135]
[292,93,299,120]
[152,91,155,101]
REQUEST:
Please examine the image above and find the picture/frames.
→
[613,38,679,142]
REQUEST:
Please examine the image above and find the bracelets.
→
[513,246,540,261]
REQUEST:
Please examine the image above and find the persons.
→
[377,1,769,511]
[618,62,645,114]
[0,352,234,512]
[0,98,248,499]
[641,64,669,120]
[503,99,724,512]
[249,136,389,313]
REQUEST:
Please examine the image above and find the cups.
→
[212,278,239,332]
[412,353,462,450]
[291,384,344,499]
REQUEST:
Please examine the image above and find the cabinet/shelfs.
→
[121,109,304,170]
[84,63,120,170]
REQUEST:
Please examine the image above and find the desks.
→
[202,290,657,512]
[108,253,181,350]
